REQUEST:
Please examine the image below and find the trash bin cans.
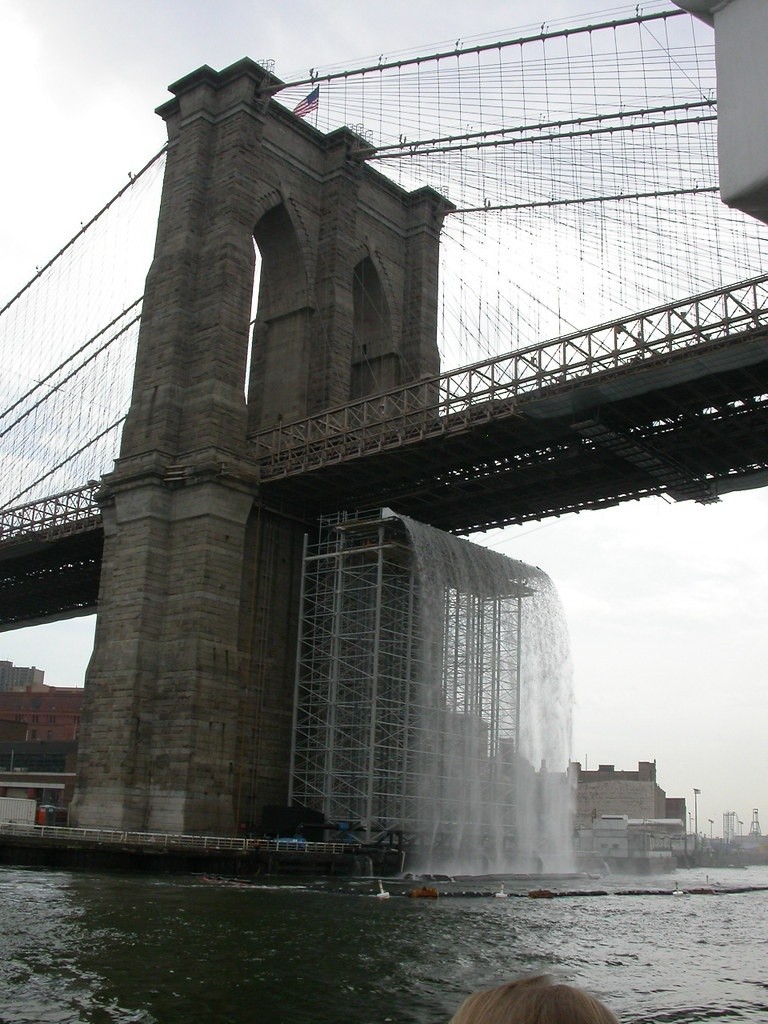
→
[36,805,52,826]
[45,807,68,827]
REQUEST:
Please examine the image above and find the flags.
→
[293,86,319,117]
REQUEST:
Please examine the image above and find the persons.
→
[450,975,618,1024]
